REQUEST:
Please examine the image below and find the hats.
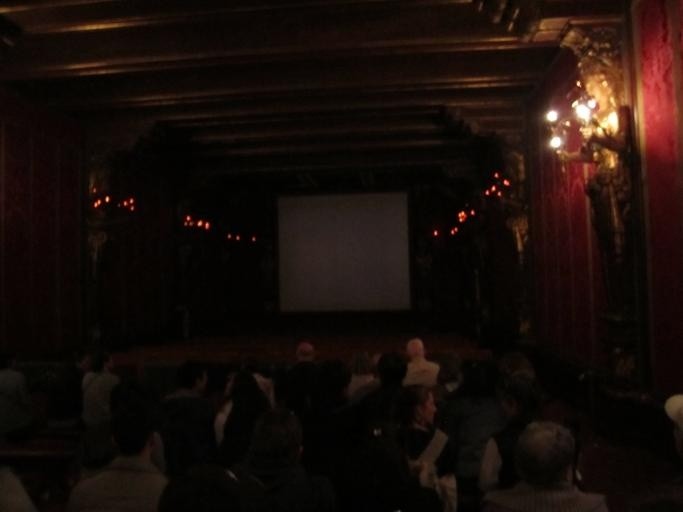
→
[664,391,683,429]
[293,341,314,356]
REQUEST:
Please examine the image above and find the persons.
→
[551,54,641,331]
[1,329,616,512]
